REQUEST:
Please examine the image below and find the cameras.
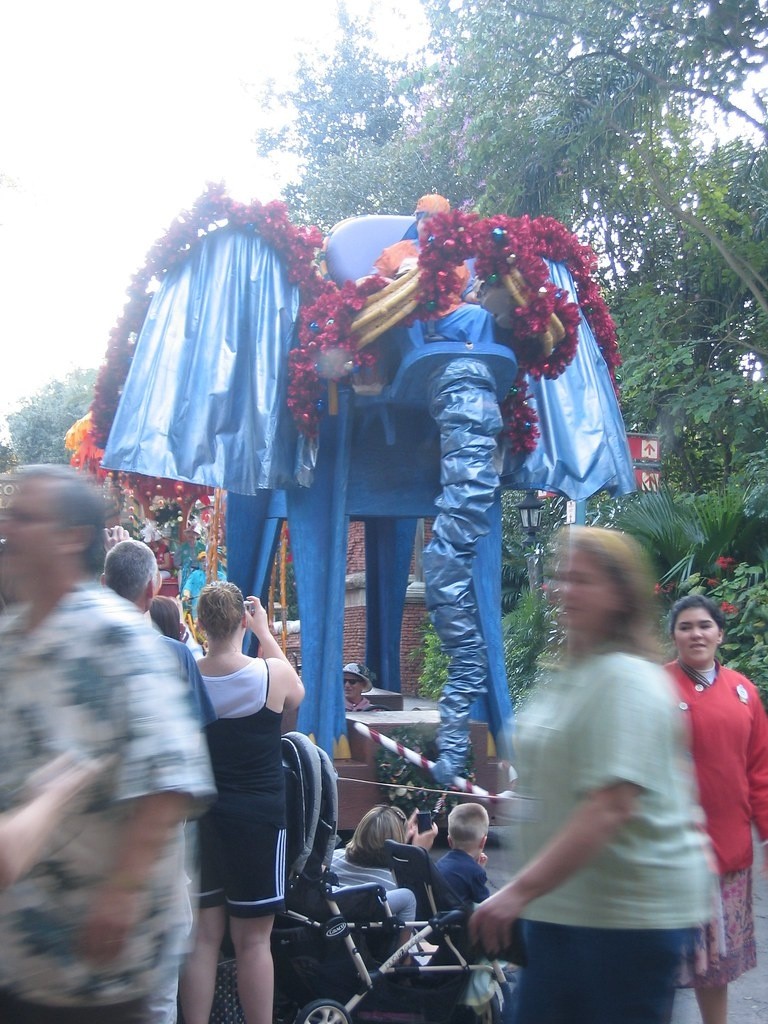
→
[416,811,432,834]
[244,601,251,616]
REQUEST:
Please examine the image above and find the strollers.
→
[219,731,520,1024]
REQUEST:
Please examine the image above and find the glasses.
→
[375,804,406,825]
[344,679,362,685]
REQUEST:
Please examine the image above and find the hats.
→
[344,662,372,693]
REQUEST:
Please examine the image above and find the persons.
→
[1,463,308,1024]
[344,660,385,712]
[663,594,768,1019]
[467,527,722,1023]
[330,803,489,989]
